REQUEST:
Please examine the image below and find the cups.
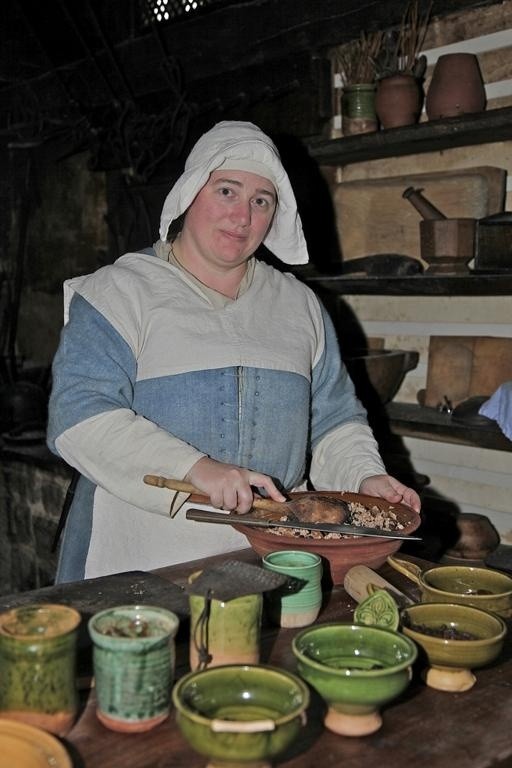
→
[88,604,181,734]
[0,605,83,741]
[188,570,264,673]
[262,549,324,629]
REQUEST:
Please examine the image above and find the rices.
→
[264,501,412,539]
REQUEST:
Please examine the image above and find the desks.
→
[0,547,511,768]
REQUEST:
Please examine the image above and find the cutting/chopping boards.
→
[0,569,189,678]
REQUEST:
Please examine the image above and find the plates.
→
[0,717,74,768]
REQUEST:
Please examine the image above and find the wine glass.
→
[172,662,311,768]
[292,589,418,737]
[367,582,507,692]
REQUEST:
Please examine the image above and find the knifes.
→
[186,507,423,540]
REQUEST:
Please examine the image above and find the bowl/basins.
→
[229,491,421,585]
[340,347,420,411]
[386,555,511,620]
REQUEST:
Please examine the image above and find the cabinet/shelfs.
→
[294,105,510,459]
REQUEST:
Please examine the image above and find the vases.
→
[372,53,487,129]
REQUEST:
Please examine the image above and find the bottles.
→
[376,73,420,129]
[340,82,378,136]
[426,53,487,121]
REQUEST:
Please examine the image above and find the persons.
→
[47,122,420,584]
